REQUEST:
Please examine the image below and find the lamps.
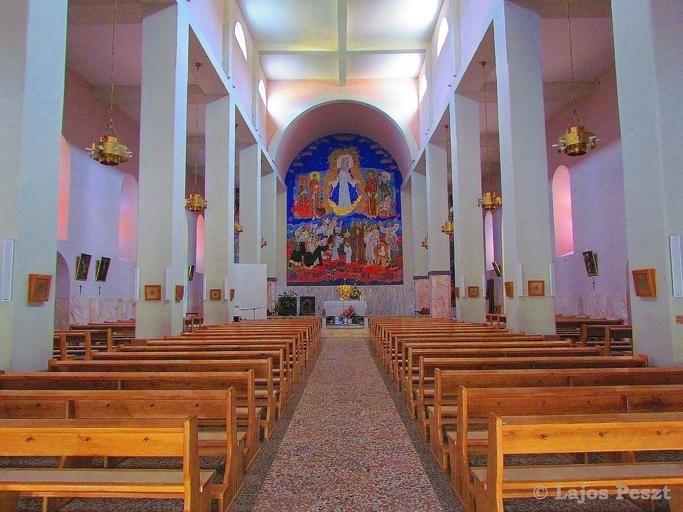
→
[84,1,131,165]
[419,60,502,250]
[548,1,599,156]
[186,60,243,234]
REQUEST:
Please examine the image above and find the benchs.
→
[0,316,321,508]
[366,313,683,511]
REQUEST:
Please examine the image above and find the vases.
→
[342,318,352,324]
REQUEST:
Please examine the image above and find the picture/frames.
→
[28,273,52,300]
[583,251,600,276]
[144,284,184,302]
[492,262,501,277]
[210,288,234,300]
[504,280,545,297]
[76,253,111,281]
[454,286,480,297]
[631,268,655,296]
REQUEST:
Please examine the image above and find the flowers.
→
[338,305,356,318]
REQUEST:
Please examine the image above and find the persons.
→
[289,156,399,270]
